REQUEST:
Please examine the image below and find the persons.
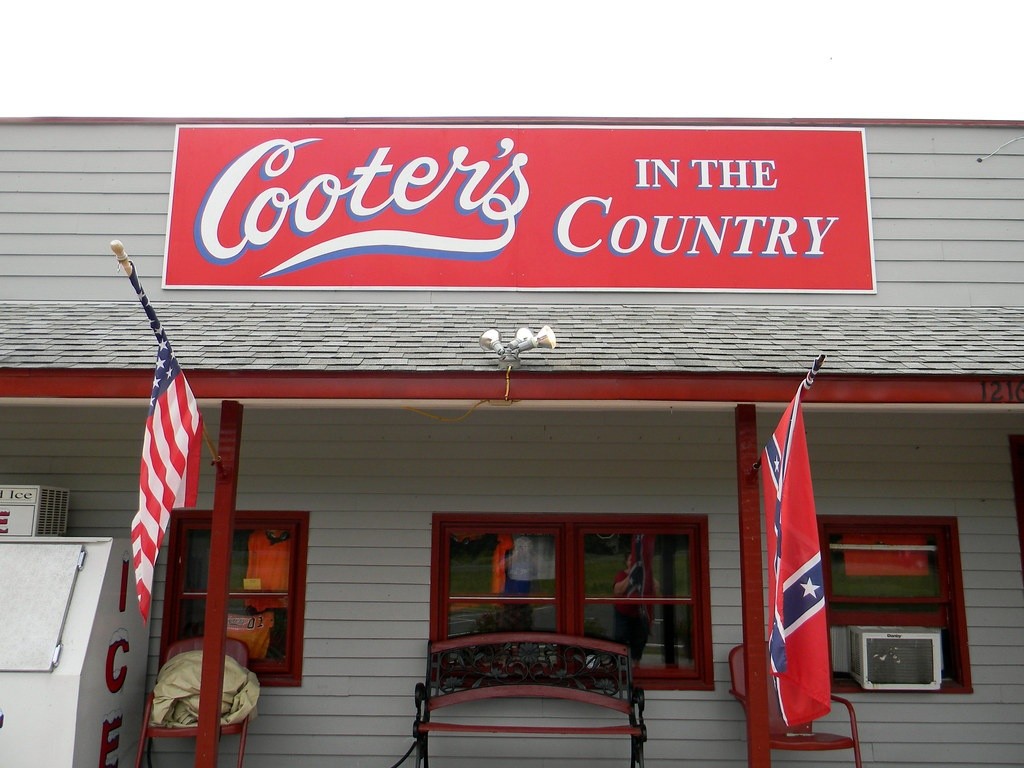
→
[613,552,661,667]
[496,537,538,632]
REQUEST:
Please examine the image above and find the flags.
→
[758,358,831,729]
[117,260,204,626]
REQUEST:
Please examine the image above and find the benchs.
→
[413,632,647,768]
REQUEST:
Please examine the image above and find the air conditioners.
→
[847,626,942,690]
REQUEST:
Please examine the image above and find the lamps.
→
[480,325,556,371]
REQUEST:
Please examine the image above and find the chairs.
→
[133,637,248,768]
[728,641,864,768]
